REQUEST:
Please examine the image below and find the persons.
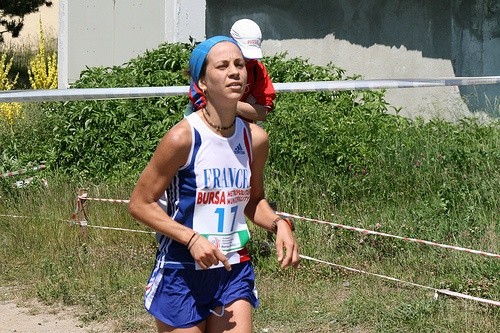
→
[184,18,277,125]
[127,36,301,333]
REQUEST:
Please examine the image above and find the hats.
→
[230,18,263,59]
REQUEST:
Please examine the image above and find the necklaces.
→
[202,108,237,132]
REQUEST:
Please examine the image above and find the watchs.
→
[270,216,296,234]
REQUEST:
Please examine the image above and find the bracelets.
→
[185,231,200,251]
